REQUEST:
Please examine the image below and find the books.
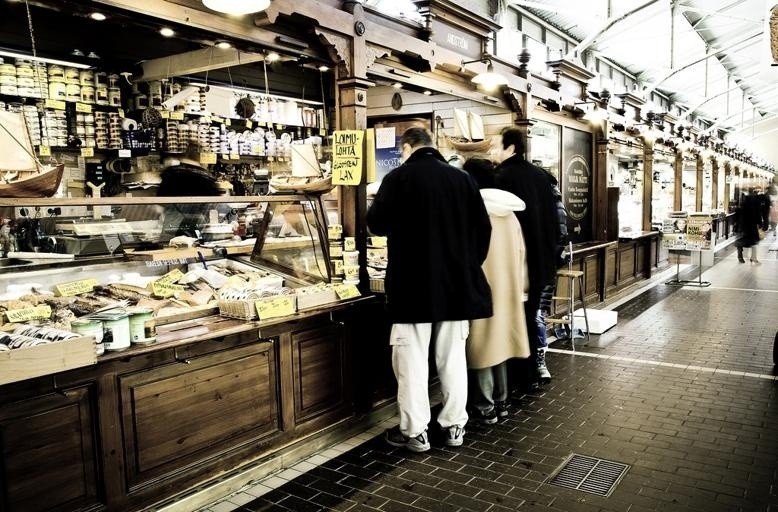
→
[83,297,133,321]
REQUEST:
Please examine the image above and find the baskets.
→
[368,278,384,292]
[220,293,295,320]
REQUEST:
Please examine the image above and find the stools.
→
[545,269,591,349]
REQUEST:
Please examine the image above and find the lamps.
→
[573,101,610,124]
[461,58,509,91]
[694,144,713,157]
[669,135,690,150]
[633,122,660,139]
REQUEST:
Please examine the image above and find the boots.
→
[534,345,551,381]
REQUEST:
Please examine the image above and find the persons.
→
[462,157,531,426]
[493,125,558,394]
[156,144,220,242]
[732,176,778,264]
[537,170,568,380]
[368,126,492,453]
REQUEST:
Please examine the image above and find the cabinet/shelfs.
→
[0,194,335,345]
[1,47,324,168]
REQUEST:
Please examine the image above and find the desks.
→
[663,245,712,287]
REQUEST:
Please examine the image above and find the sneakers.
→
[470,410,497,425]
[383,426,431,452]
[445,426,465,447]
[494,401,508,417]
